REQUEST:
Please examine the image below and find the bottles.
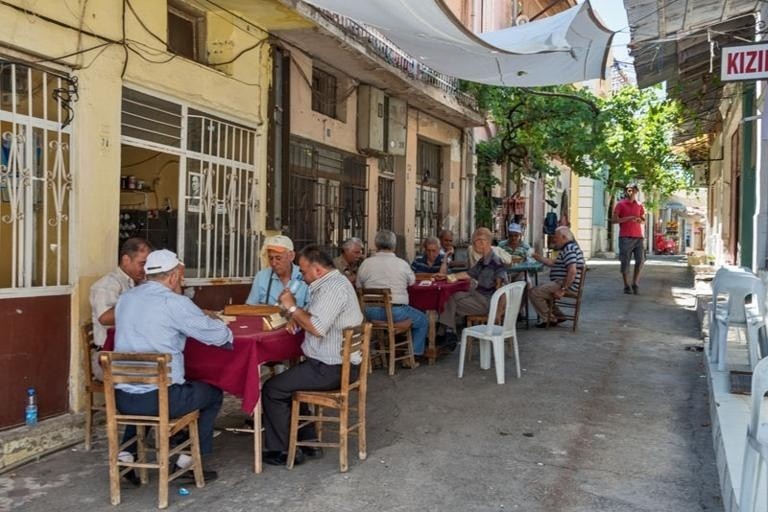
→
[24,387,38,426]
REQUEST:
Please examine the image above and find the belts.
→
[369,304,405,307]
[90,343,104,351]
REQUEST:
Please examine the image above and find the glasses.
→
[472,239,486,244]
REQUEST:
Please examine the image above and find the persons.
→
[439,230,465,271]
[411,236,450,280]
[531,225,585,329]
[467,228,514,267]
[356,229,429,364]
[433,227,509,353]
[612,182,646,294]
[88,237,152,384]
[244,235,311,311]
[111,249,234,491]
[498,223,535,323]
[331,236,364,285]
[260,244,364,467]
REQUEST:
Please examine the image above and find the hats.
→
[264,235,294,253]
[508,223,522,234]
[143,249,185,275]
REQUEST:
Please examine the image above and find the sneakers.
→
[300,443,324,459]
[403,355,420,368]
[109,459,141,489]
[537,322,557,328]
[552,318,567,326]
[261,448,304,465]
[227,419,265,433]
[624,287,632,293]
[211,429,224,439]
[171,465,218,485]
[632,284,640,295]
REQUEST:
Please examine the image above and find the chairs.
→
[358,287,416,375]
[286,322,372,472]
[547,267,587,331]
[739,356,767,512]
[711,272,766,372]
[79,319,104,452]
[99,352,205,509]
[467,280,513,362]
[708,269,759,355]
[457,281,527,384]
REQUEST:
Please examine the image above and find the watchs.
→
[287,305,298,317]
